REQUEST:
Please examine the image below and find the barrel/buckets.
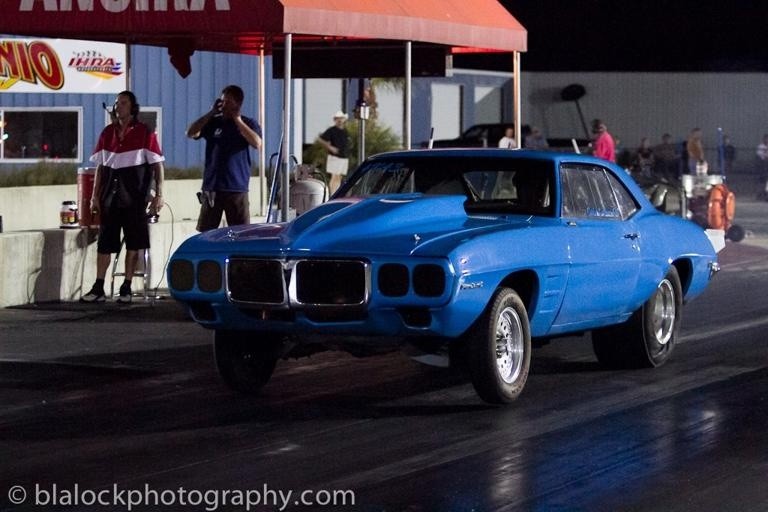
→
[75,167,101,229]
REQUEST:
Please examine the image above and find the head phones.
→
[112,90,138,116]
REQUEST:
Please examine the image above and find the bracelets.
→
[156,192,162,197]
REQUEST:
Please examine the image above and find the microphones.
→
[102,101,111,115]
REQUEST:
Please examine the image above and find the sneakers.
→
[117,291,133,303]
[79,291,107,304]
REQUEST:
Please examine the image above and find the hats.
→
[332,110,349,119]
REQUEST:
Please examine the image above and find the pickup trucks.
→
[422,121,595,151]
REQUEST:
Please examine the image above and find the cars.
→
[166,146,719,402]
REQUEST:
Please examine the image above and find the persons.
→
[524,125,551,150]
[715,135,737,172]
[654,132,684,182]
[497,125,518,149]
[184,83,265,235]
[636,137,656,179]
[316,111,355,196]
[584,118,618,163]
[510,166,549,207]
[686,126,707,175]
[752,132,768,196]
[76,89,166,304]
